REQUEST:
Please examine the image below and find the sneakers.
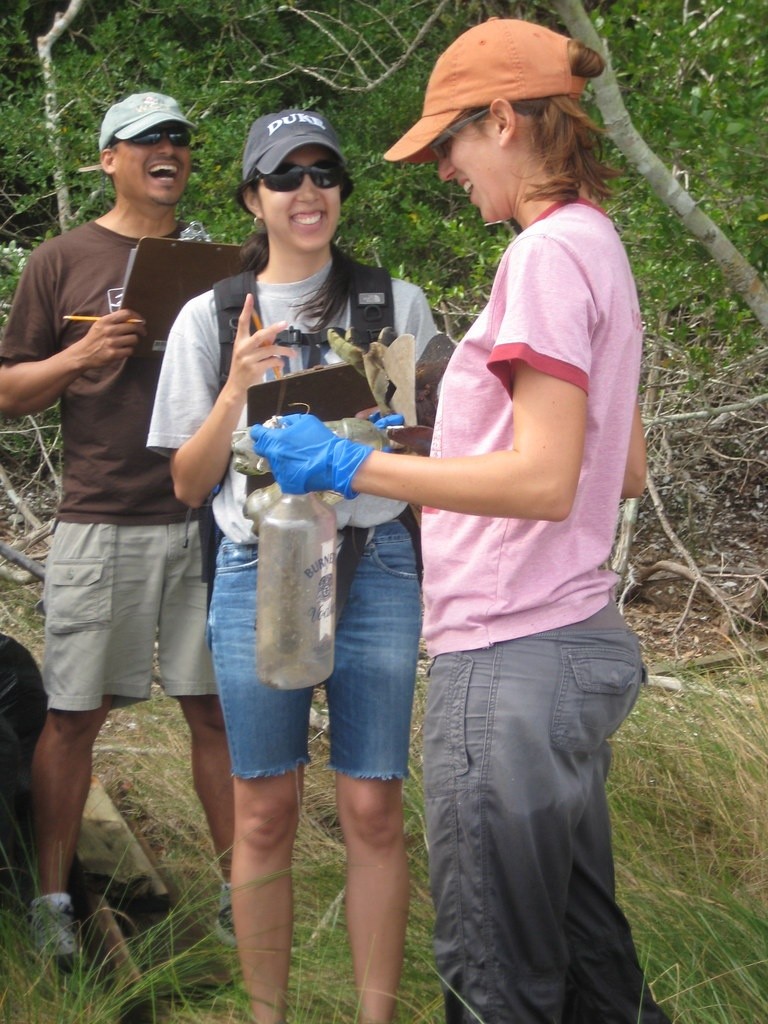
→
[26,901,78,969]
[213,884,240,946]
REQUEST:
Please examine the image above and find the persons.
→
[146,107,462,1024]
[248,14,677,1024]
[0,81,234,982]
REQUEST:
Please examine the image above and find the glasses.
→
[429,107,490,160]
[250,161,344,192]
[109,127,192,147]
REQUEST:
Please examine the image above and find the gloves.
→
[325,325,400,415]
[249,412,375,501]
[366,411,404,453]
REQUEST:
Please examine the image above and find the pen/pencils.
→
[252,307,281,379]
[62,315,143,322]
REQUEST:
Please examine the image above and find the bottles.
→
[231,417,390,475]
[255,489,338,690]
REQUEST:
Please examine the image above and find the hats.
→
[242,109,345,181]
[383,14,587,164]
[99,92,197,151]
